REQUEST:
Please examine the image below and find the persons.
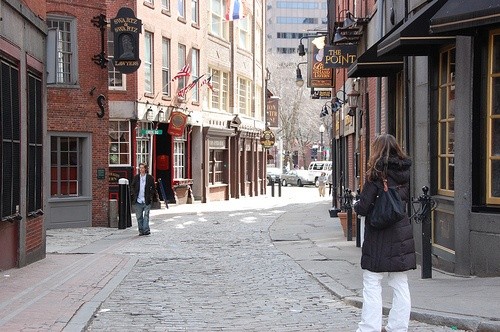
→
[353,133,418,332]
[130,162,155,236]
[328,172,332,197]
[318,172,327,198]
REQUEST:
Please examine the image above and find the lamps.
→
[320,97,345,118]
[158,108,164,123]
[347,83,365,114]
[146,106,153,121]
[296,36,308,57]
[332,9,371,43]
[296,62,308,87]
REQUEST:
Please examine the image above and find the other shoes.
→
[139,231,143,236]
[143,231,150,235]
[382,325,387,332]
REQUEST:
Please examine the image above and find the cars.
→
[266,167,282,186]
[281,169,320,187]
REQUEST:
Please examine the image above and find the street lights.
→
[319,124,325,161]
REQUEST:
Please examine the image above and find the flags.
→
[171,64,191,81]
[200,75,215,93]
[178,74,204,98]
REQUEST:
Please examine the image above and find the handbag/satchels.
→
[365,160,405,230]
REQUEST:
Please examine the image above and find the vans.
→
[308,160,333,185]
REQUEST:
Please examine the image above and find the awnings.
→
[347,20,404,78]
[429,0,500,36]
[376,0,456,58]
